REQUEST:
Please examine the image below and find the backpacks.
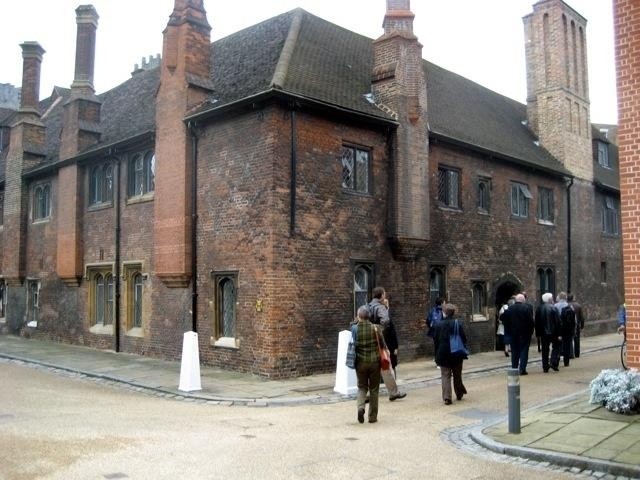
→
[540,304,557,334]
[560,303,575,334]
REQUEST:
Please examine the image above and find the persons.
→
[617,304,626,334]
[380,299,398,384]
[349,304,392,424]
[426,297,446,370]
[355,286,407,404]
[432,304,469,406]
[497,290,585,375]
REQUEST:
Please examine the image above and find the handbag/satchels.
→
[346,326,359,367]
[373,324,392,370]
[449,318,470,355]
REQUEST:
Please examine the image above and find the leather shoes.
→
[365,396,369,402]
[369,417,377,422]
[389,393,406,401]
[505,348,512,356]
[519,369,528,375]
[444,393,465,404]
[537,349,579,373]
[357,409,364,423]
[512,365,517,369]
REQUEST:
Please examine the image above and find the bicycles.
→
[618,326,628,370]
